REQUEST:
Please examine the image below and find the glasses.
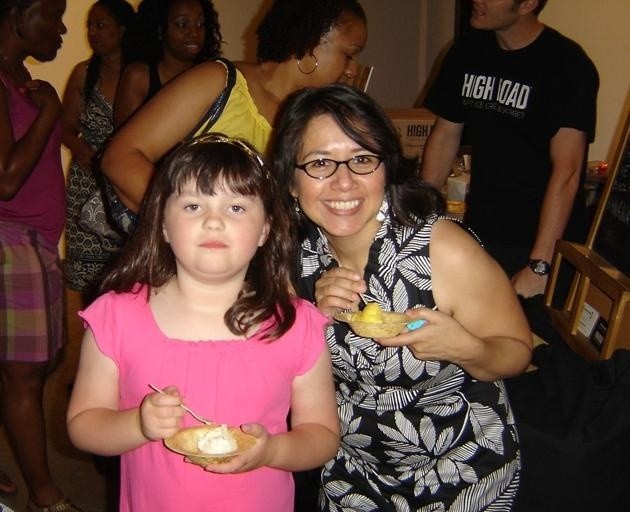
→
[293,156,382,180]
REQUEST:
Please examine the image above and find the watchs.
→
[525,258,552,275]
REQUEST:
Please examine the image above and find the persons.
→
[269,85,532,512]
[0,0,84,512]
[0,471,18,497]
[67,133,340,512]
[113,0,228,134]
[100,0,367,215]
[419,0,600,300]
[60,0,136,333]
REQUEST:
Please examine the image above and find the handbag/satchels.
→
[93,56,236,238]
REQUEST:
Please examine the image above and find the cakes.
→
[348,302,386,323]
[197,424,238,455]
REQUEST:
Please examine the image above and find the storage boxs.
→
[384,107,440,161]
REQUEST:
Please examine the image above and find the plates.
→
[333,311,415,340]
[164,425,258,467]
